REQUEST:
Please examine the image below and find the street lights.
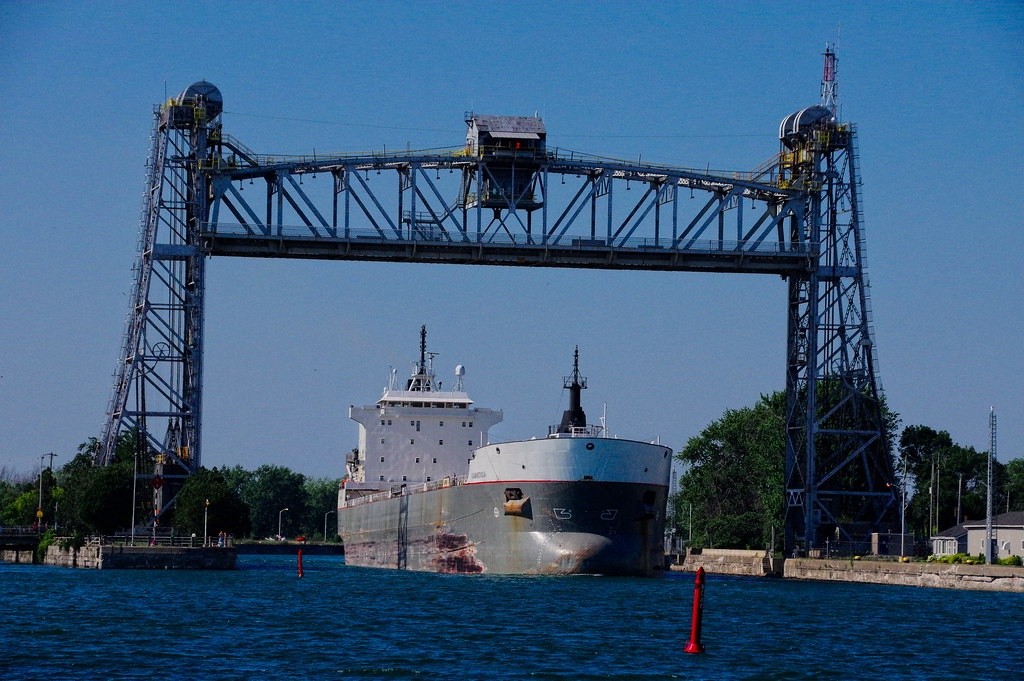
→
[323,511,333,542]
[279,507,289,540]
[37,453,45,528]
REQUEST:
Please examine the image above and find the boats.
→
[337,323,675,578]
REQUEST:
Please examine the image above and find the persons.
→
[218,531,223,542]
[452,473,457,486]
[224,533,228,546]
